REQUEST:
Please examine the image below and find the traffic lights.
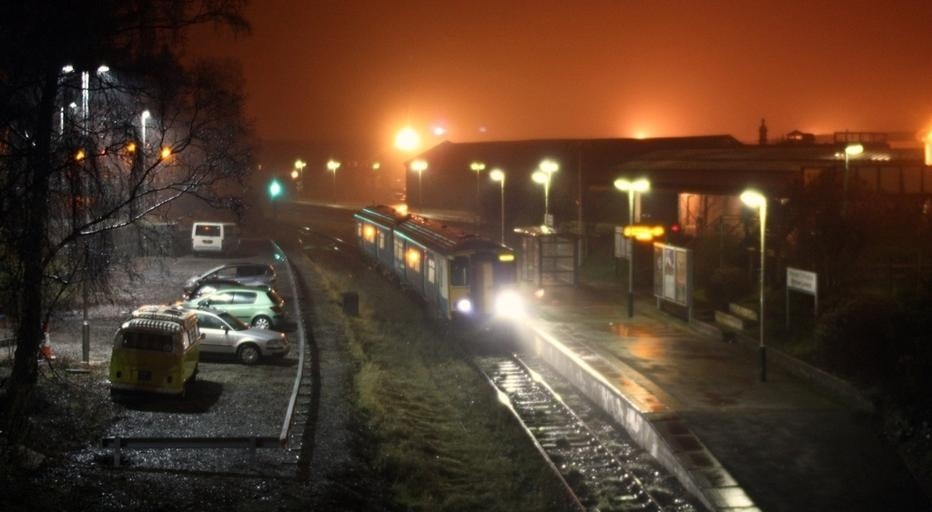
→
[269,179,282,200]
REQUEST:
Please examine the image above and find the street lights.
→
[59,64,173,270]
[842,143,864,216]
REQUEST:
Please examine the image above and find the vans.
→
[192,222,239,257]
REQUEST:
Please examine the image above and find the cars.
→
[108,261,291,400]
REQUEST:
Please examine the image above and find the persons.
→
[39,324,54,360]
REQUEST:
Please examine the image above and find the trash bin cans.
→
[342,293,358,317]
